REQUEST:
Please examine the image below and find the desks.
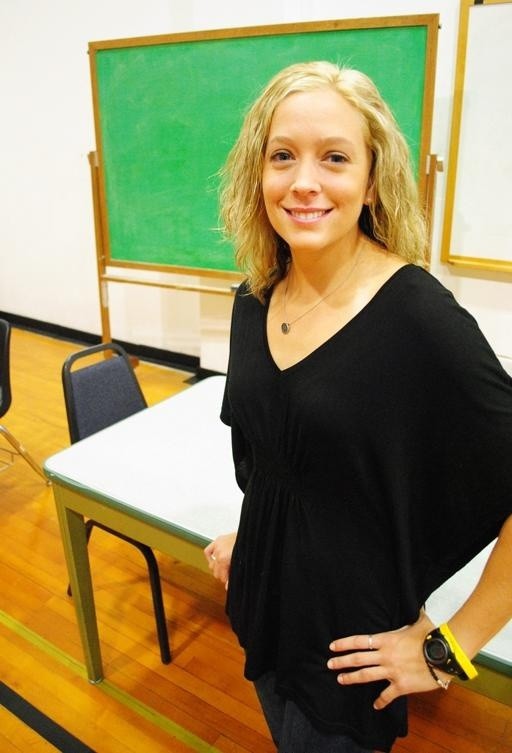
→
[42,374,512,709]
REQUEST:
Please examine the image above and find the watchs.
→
[422,621,479,692]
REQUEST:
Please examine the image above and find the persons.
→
[204,58,512,753]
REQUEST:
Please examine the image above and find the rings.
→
[209,555,216,561]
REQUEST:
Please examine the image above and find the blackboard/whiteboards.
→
[441,2,512,281]
[89,14,438,283]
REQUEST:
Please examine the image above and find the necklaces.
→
[280,238,368,336]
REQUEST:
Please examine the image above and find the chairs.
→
[1,318,20,424]
[60,342,174,666]
